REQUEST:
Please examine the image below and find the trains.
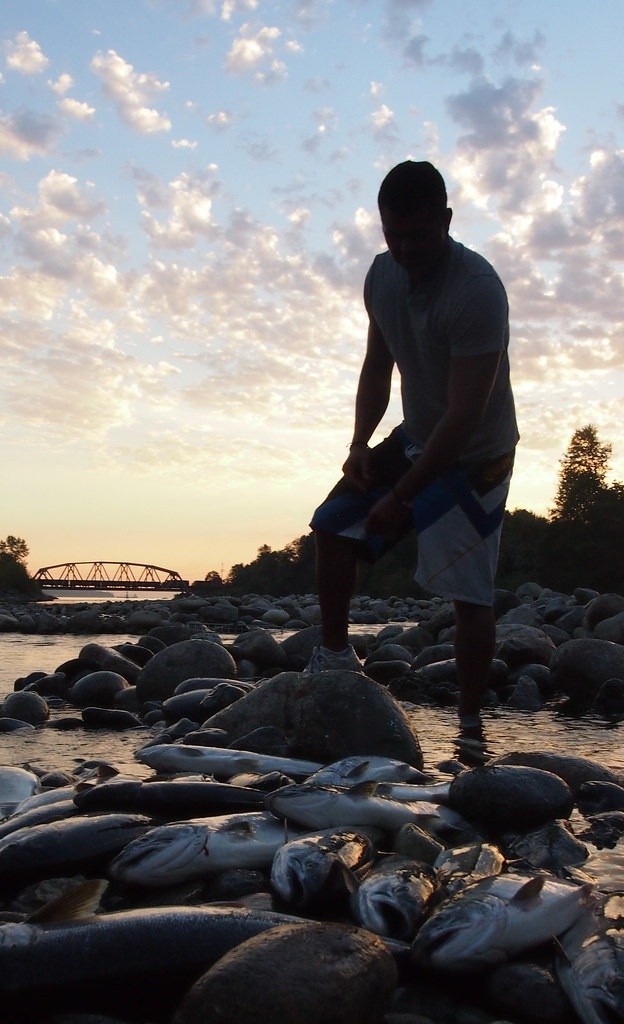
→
[36,579,191,591]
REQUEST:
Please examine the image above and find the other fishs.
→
[0,743,624,1024]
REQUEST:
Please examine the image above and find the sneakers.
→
[302,645,361,675]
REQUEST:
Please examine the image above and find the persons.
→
[300,160,522,766]
